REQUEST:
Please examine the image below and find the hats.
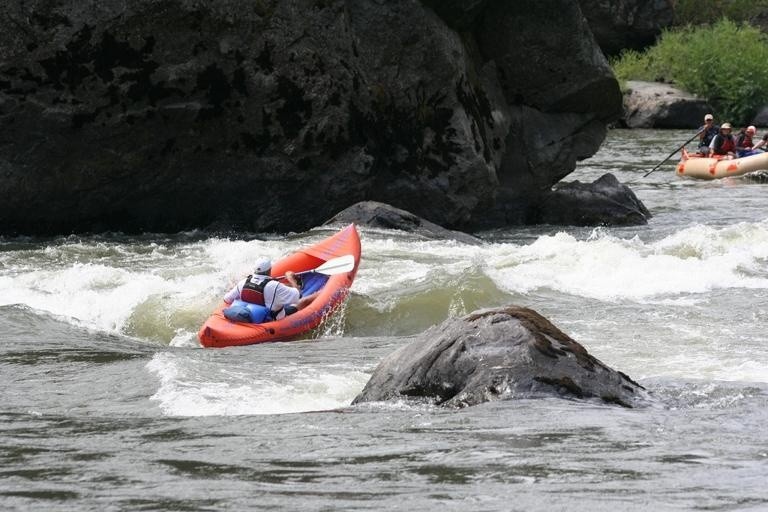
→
[253,257,272,272]
[747,125,756,135]
[719,121,732,134]
[704,114,713,120]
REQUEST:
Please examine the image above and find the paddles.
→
[274,254,355,281]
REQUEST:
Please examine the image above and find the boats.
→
[198,220,361,348]
[677,146,767,180]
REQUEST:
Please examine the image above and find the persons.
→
[696,114,768,161]
[221,254,324,323]
